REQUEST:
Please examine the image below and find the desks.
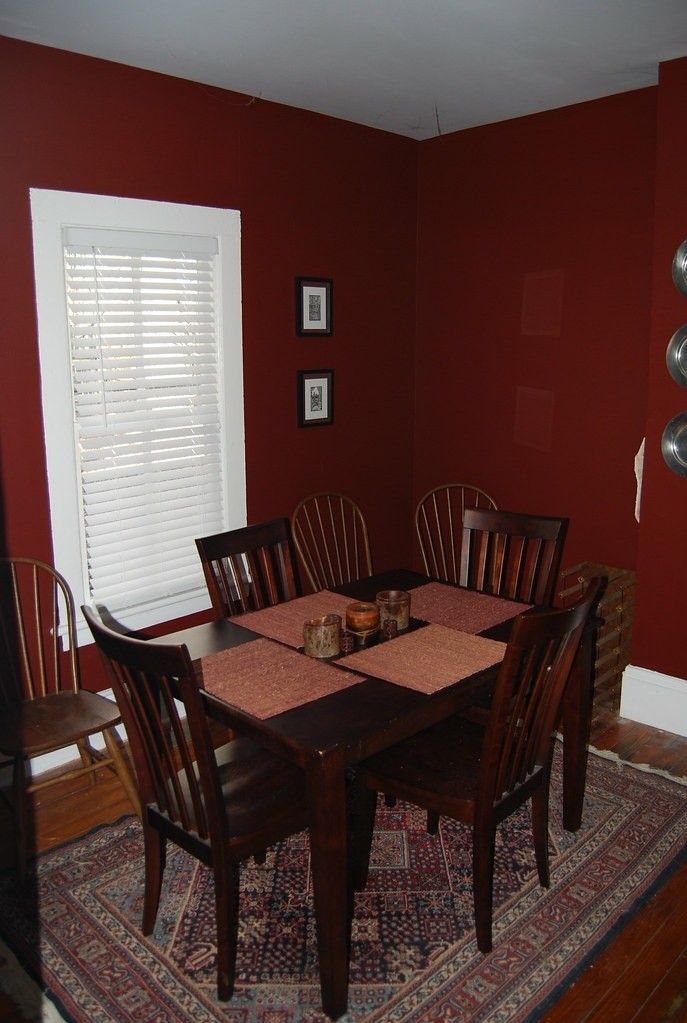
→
[145,569,605,1020]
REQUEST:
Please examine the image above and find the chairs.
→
[415,483,500,585]
[1,557,142,824]
[292,491,372,593]
[457,507,571,607]
[355,575,599,952]
[81,605,362,1002]
[194,516,397,807]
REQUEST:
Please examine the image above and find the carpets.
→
[1,708,686,1021]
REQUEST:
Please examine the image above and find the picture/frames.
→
[297,368,334,428]
[294,276,333,337]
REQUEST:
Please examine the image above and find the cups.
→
[345,600,380,646]
[303,614,341,658]
[376,589,411,631]
